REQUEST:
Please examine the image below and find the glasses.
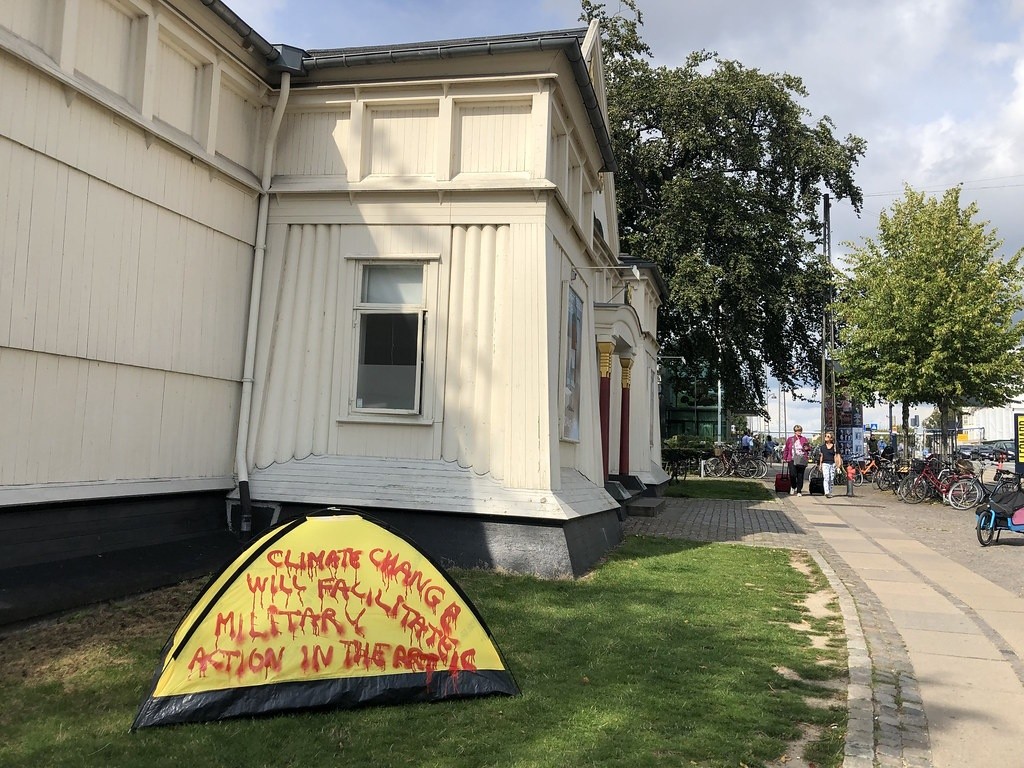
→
[825,435,832,438]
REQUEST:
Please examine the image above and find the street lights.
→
[767,393,777,435]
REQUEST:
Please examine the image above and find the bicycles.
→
[662,440,769,485]
[808,448,1024,511]
[773,447,783,462]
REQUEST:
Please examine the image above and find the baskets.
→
[714,448,721,456]
[910,458,926,474]
[762,450,768,457]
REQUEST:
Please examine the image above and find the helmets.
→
[880,436,883,439]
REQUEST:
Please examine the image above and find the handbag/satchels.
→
[834,444,842,468]
[794,454,808,467]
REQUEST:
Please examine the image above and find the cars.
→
[994,442,1016,461]
[971,447,995,460]
[807,443,822,464]
[957,445,975,458]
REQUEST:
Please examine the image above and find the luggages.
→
[809,467,825,496]
[775,461,791,494]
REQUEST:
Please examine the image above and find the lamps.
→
[658,356,686,365]
[571,265,641,281]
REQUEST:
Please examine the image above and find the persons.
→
[819,431,841,498]
[763,435,777,468]
[782,425,812,497]
[742,430,761,457]
[877,436,887,455]
[866,434,880,456]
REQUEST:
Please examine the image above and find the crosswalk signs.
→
[871,424,878,430]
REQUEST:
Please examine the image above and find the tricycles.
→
[974,491,1024,546]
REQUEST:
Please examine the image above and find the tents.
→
[126,505,519,730]
